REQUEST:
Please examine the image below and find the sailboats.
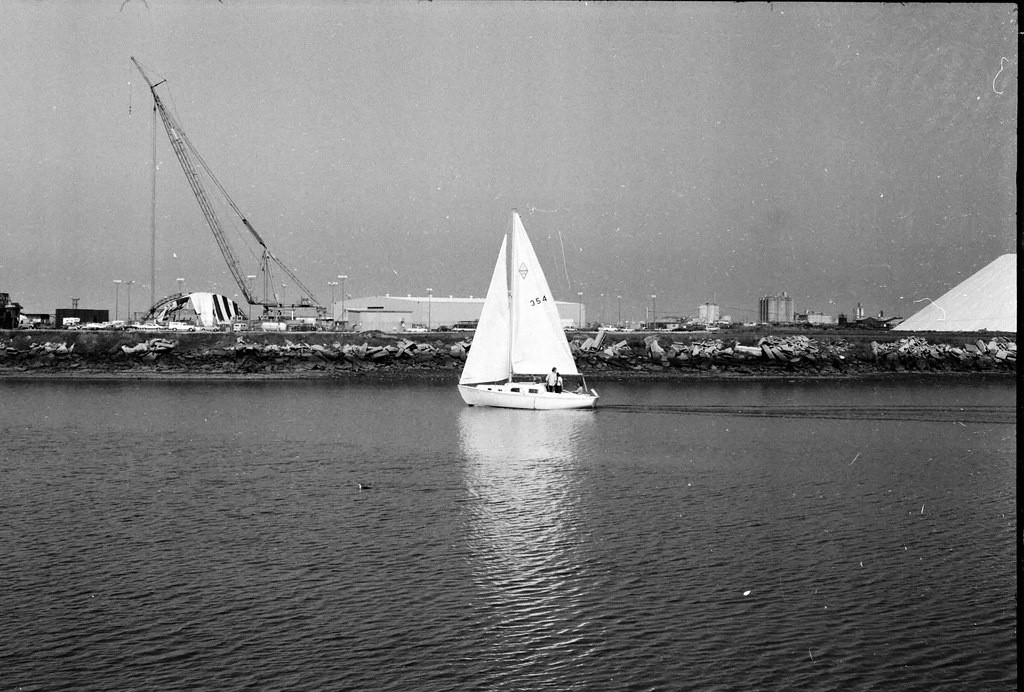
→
[456,207,602,410]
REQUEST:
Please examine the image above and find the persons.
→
[554,373,563,393]
[574,381,583,395]
[546,367,557,392]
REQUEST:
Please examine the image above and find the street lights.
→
[124,279,136,321]
[113,279,122,320]
[651,294,657,330]
[327,273,347,329]
[577,291,584,330]
[426,288,434,332]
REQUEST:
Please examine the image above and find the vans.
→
[233,322,248,331]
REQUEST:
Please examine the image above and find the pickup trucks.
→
[172,322,196,332]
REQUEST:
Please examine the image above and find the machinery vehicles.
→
[124,54,328,322]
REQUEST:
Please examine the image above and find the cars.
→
[597,324,618,332]
[563,325,577,331]
[200,324,221,331]
[18,319,34,329]
